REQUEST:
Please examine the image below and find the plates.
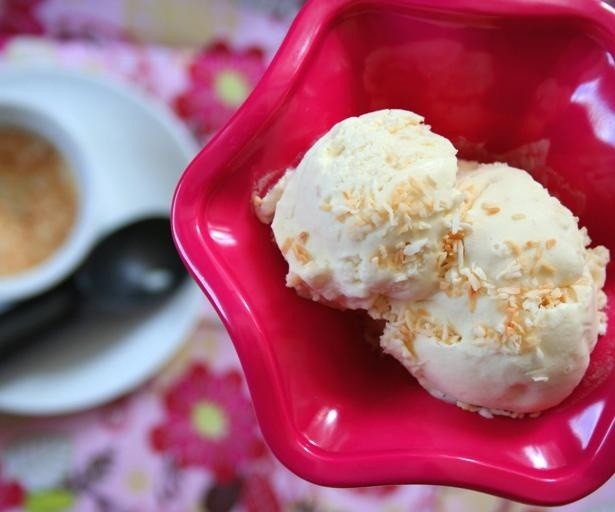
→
[0,63,201,417]
[169,1,614,507]
